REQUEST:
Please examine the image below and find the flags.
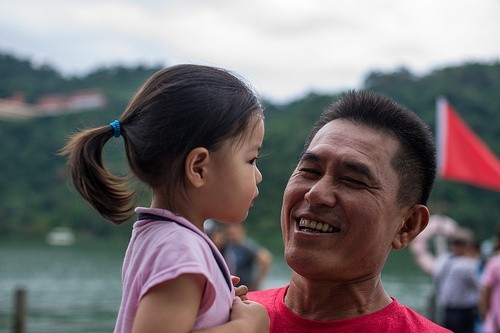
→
[438,97,500,191]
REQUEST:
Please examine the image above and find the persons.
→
[432,232,500,333]
[203,220,271,289]
[241,89,453,333]
[55,64,265,333]
